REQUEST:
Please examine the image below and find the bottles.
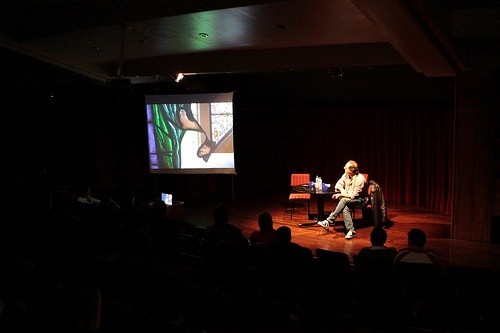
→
[318,177,322,191]
[315,176,319,188]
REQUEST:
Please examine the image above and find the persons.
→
[317,160,370,239]
[0,186,449,333]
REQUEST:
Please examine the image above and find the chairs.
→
[289,174,311,220]
[351,173,368,220]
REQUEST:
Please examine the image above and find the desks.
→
[292,183,345,221]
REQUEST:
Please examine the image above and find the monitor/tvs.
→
[161,193,173,208]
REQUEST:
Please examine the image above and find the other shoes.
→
[345,229,357,238]
[318,220,329,229]
